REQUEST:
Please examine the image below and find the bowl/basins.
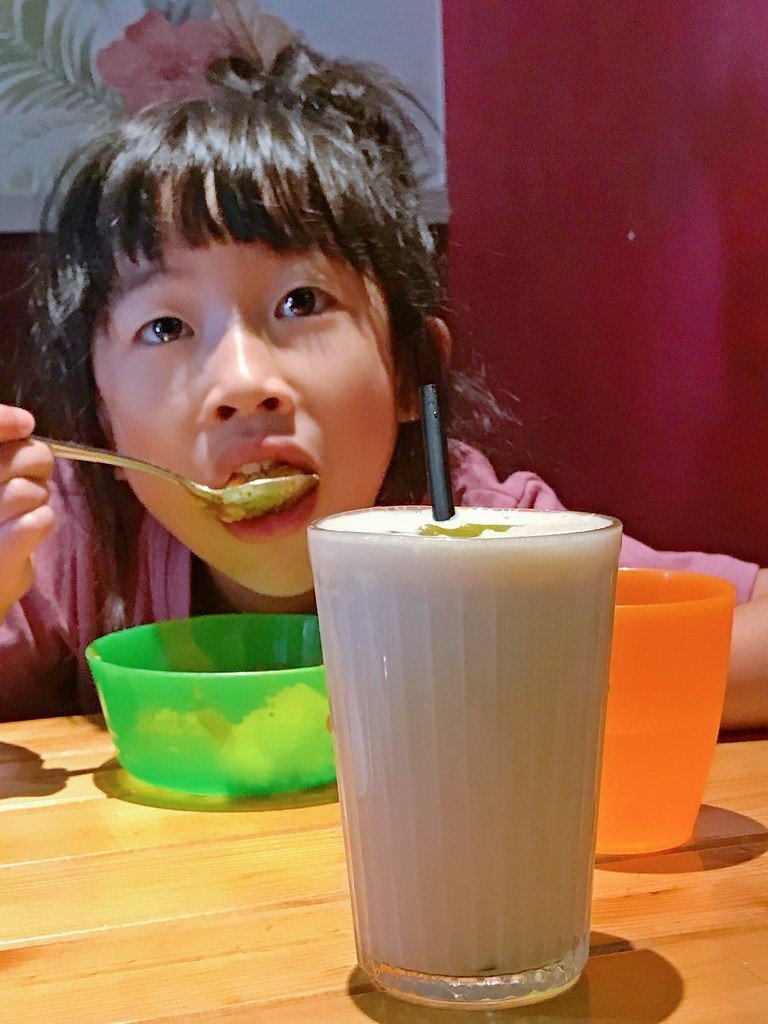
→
[85,612,338,797]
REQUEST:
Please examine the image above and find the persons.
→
[0,41,767,731]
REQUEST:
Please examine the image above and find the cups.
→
[303,503,623,1011]
[593,565,736,852]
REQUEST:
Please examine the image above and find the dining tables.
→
[2,715,768,1024]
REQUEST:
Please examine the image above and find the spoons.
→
[29,432,318,524]
